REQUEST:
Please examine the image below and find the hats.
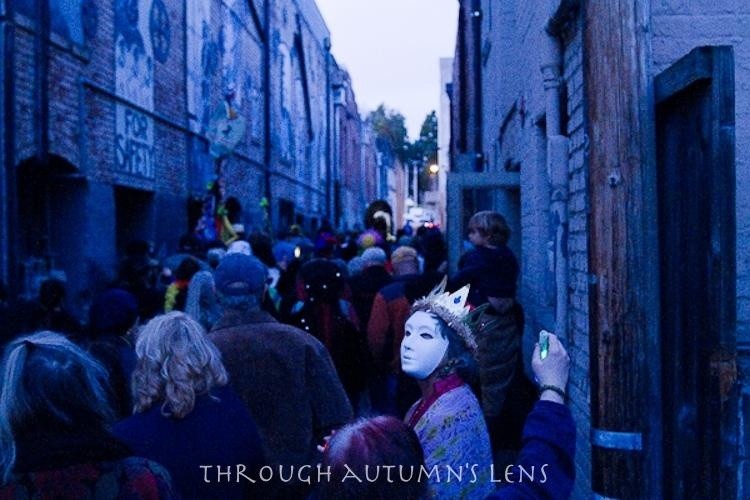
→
[89,288,137,333]
[214,257,266,294]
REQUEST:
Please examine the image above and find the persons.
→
[302,208,523,330]
[199,221,303,303]
[204,253,355,499]
[1,330,173,500]
[0,238,196,335]
[316,331,572,500]
[400,276,497,499]
[109,311,267,500]
